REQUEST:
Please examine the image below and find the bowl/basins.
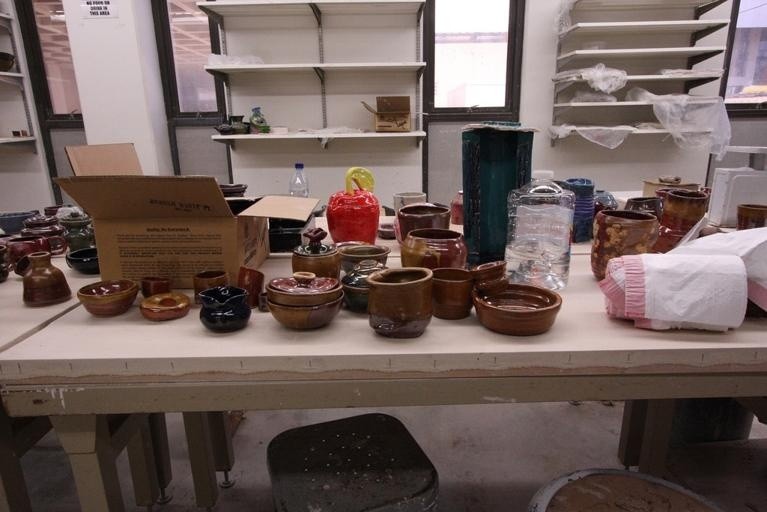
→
[338,241,391,271]
[265,271,344,306]
[77,278,139,316]
[0,210,36,237]
[472,261,506,285]
[472,279,563,336]
[65,247,100,275]
[264,291,347,330]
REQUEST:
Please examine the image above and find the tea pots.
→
[7,235,68,266]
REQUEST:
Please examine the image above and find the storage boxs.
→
[361,96,413,132]
[51,143,320,290]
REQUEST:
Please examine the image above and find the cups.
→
[393,191,427,242]
[239,264,266,310]
[193,270,230,304]
[142,277,173,298]
[429,266,475,320]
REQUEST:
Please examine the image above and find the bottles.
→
[503,169,576,292]
[450,189,464,225]
[288,163,309,198]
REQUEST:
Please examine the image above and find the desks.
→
[0,253,767,512]
[0,237,101,351]
[701,223,767,311]
[270,217,598,257]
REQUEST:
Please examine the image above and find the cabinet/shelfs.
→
[704,146,767,192]
[550,0,730,149]
[0,0,59,236]
[196,0,435,215]
